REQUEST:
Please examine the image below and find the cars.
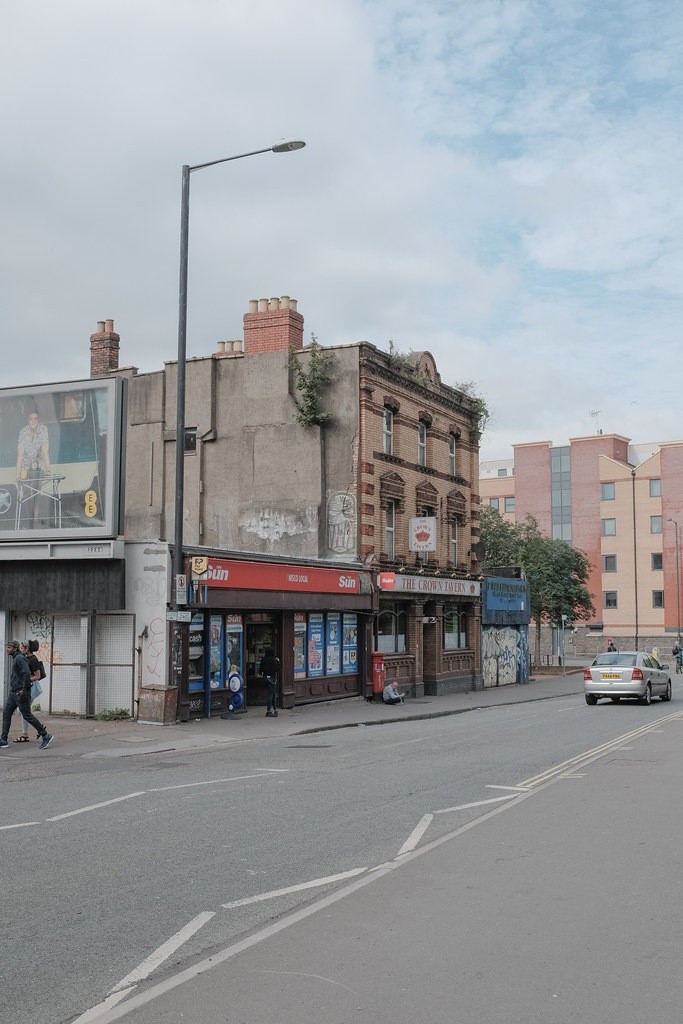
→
[584,650,672,706]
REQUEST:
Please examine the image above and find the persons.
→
[382,681,405,706]
[263,647,282,716]
[0,640,53,749]
[15,411,51,529]
[673,641,682,669]
[607,643,615,652]
[12,640,46,742]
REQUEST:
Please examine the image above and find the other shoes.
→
[274,711,278,717]
[266,711,273,717]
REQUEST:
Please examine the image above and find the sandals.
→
[35,724,46,739]
[12,736,28,742]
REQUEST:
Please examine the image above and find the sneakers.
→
[39,735,53,750]
[0,737,9,748]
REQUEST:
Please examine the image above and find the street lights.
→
[168,140,307,725]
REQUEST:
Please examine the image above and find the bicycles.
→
[673,647,683,674]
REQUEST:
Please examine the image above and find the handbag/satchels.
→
[37,661,45,681]
